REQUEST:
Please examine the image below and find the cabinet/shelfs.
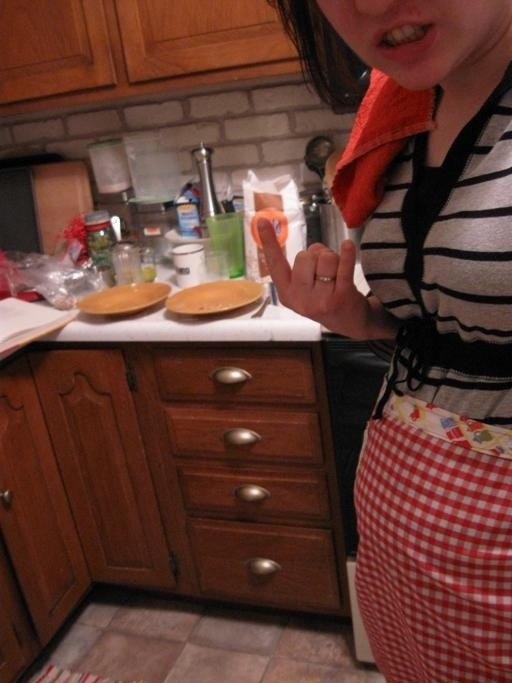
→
[144,344,343,612]
[28,345,179,594]
[0,351,92,652]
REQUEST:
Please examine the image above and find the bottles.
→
[84,137,247,289]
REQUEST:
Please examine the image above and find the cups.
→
[316,196,363,265]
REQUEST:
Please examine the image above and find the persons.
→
[255,0,512,683]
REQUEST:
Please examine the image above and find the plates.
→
[77,281,266,317]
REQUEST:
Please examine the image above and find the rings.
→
[315,274,337,282]
